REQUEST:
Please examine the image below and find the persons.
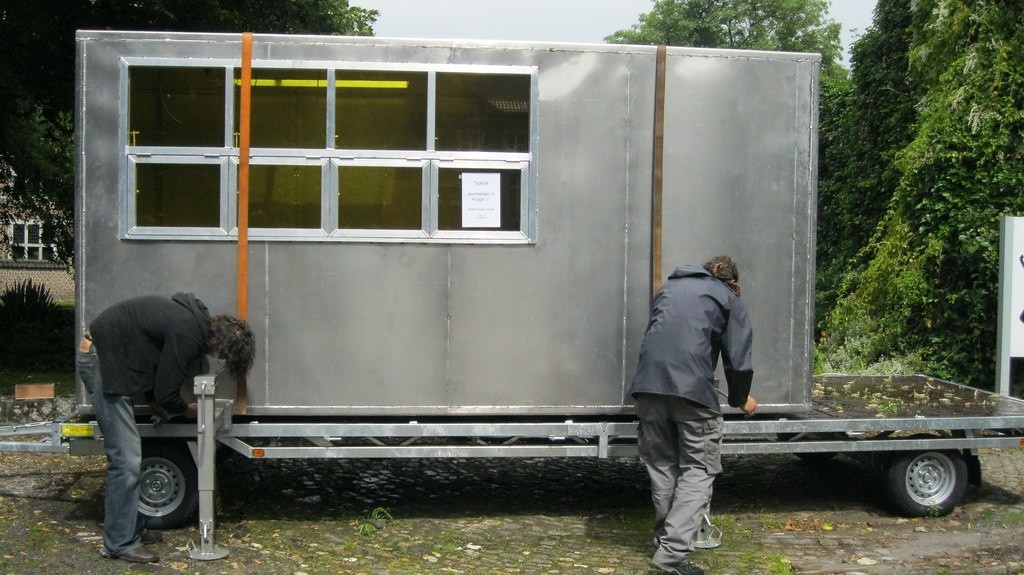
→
[77,292,257,562]
[630,254,760,575]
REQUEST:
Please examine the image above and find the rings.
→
[754,411,755,414]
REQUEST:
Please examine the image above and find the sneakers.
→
[652,520,665,537]
[651,559,704,575]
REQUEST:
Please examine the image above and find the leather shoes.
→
[141,529,163,544]
[119,545,159,563]
